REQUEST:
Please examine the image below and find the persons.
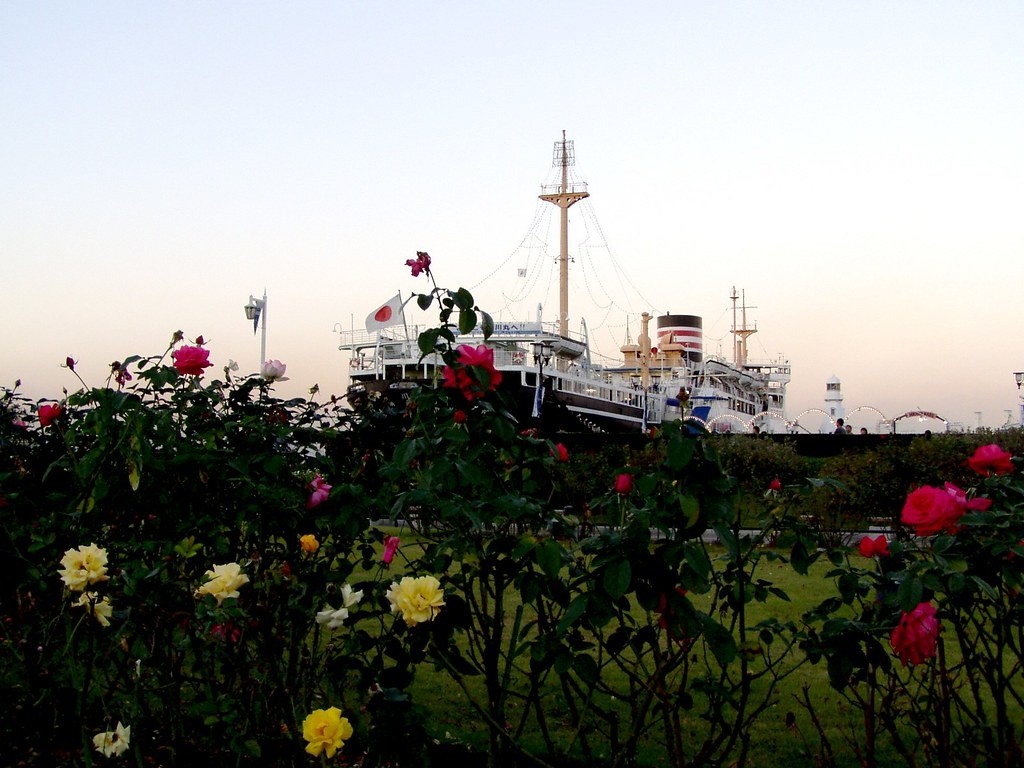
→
[834,418,846,434]
[861,428,867,434]
[762,393,768,412]
[753,426,760,433]
[653,382,659,394]
[846,425,852,434]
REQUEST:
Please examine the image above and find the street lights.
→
[244,288,269,378]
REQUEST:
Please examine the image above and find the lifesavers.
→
[348,357,361,369]
[512,351,525,364]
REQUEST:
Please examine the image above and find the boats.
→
[332,127,794,436]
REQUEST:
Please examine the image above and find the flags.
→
[365,294,404,334]
